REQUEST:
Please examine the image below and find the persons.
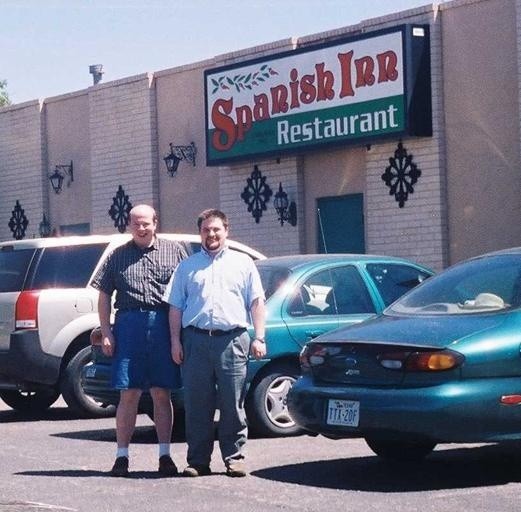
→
[161,206,266,477]
[89,205,190,478]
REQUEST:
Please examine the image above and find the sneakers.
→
[159,455,211,476]
[112,456,128,476]
[226,459,246,476]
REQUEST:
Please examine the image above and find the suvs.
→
[1,232,269,415]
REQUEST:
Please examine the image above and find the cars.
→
[82,251,473,438]
[284,246,521,470]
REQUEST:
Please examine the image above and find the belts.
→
[190,325,246,336]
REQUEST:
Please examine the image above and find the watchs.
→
[256,337,266,344]
